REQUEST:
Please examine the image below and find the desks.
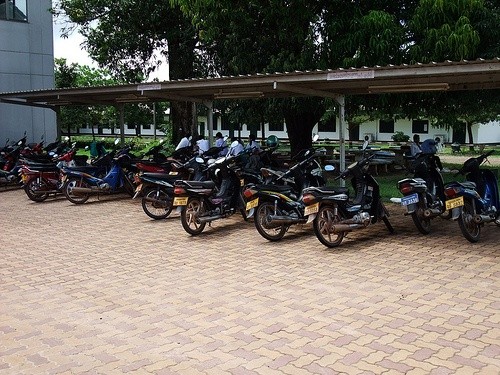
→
[309,144,411,174]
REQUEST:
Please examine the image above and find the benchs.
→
[451,144,461,153]
[238,138,262,146]
[402,146,415,168]
[264,139,291,146]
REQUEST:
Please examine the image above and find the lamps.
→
[115,96,149,102]
[45,100,72,105]
[368,82,449,94]
[214,89,264,99]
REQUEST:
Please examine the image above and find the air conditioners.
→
[434,135,444,143]
[364,133,374,142]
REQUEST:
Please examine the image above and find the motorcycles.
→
[0,130,79,203]
[172,141,250,235]
[302,140,393,248]
[389,150,453,235]
[59,138,130,204]
[440,144,500,243]
[131,149,203,221]
[126,141,170,173]
[241,134,329,241]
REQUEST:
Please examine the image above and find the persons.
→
[245,135,261,151]
[403,134,423,159]
[230,137,245,156]
[214,131,229,156]
[195,135,212,155]
[174,133,192,151]
